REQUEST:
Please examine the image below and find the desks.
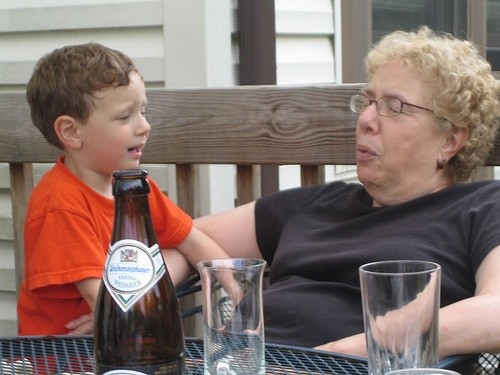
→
[0,336,370,375]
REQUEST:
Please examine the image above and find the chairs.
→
[177,264,500,375]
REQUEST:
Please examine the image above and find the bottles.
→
[94,168,186,375]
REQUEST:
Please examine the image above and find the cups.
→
[198,257,267,375]
[359,260,441,375]
[385,368,460,375]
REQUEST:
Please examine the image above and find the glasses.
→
[348,94,433,117]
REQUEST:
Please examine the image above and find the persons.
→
[7,43,245,375]
[64,26,500,375]
